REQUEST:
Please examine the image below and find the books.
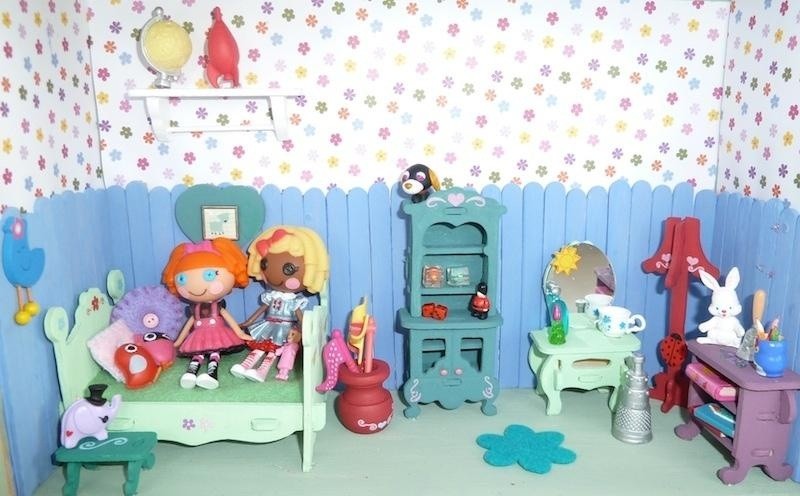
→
[685,362,738,402]
[689,402,736,438]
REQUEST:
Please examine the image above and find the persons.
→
[161,237,255,390]
[231,226,330,383]
[274,325,301,381]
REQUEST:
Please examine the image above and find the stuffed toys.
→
[471,281,490,320]
[695,267,746,349]
[58,384,124,449]
[397,163,440,204]
